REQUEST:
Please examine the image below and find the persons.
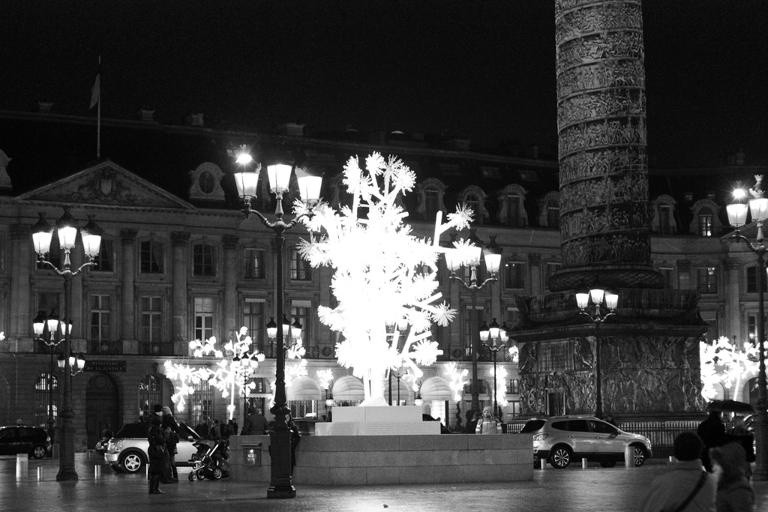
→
[267,408,301,484]
[638,402,756,512]
[147,403,267,495]
[465,406,503,434]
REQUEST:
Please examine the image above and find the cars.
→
[94,426,117,453]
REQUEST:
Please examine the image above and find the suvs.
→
[521,416,654,466]
[104,420,232,474]
[1,425,51,458]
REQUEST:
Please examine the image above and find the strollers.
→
[188,437,231,482]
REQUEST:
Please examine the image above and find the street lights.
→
[479,317,508,420]
[574,288,618,418]
[444,241,503,414]
[232,140,323,500]
[723,170,768,500]
[29,205,104,479]
[29,309,73,446]
[233,352,260,431]
[264,312,303,361]
[58,350,86,381]
